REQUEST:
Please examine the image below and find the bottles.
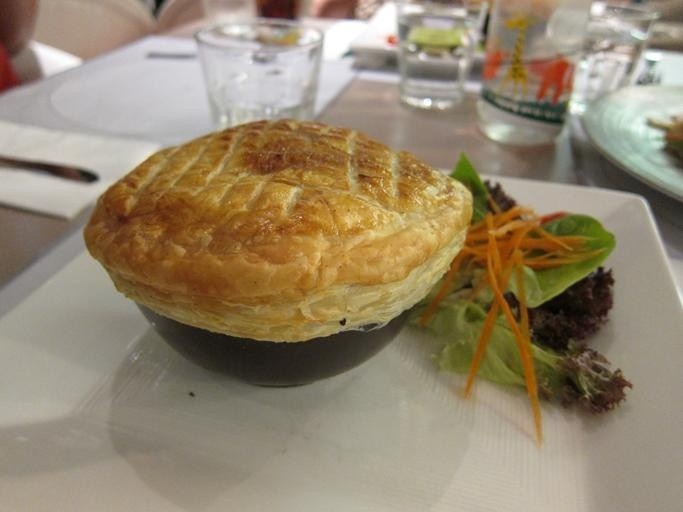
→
[474,1,591,152]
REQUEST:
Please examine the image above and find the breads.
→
[84,118,474,344]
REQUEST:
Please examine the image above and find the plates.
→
[346,1,490,72]
[583,83,682,204]
[0,169,683,512]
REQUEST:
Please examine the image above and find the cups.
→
[194,20,324,133]
[393,0,473,113]
[566,1,661,117]
